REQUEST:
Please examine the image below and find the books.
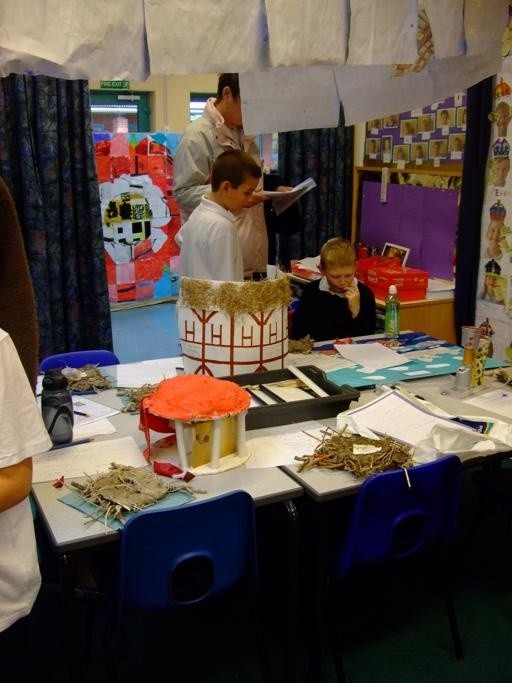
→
[72,393,120,430]
[259,177,318,215]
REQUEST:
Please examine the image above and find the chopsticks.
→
[245,382,287,404]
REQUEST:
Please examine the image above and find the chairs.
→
[284,310,296,341]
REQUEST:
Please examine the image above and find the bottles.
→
[384,284,401,340]
[41,367,77,447]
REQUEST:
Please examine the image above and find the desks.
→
[286,250,457,360]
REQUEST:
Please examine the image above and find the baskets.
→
[364,263,429,302]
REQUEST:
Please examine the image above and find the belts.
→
[244,271,267,281]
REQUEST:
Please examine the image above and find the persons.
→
[290,236,379,340]
[495,101,512,136]
[492,156,510,187]
[478,269,506,305]
[365,105,468,164]
[482,221,507,260]
[0,319,73,683]
[171,70,293,281]
[176,147,262,283]
